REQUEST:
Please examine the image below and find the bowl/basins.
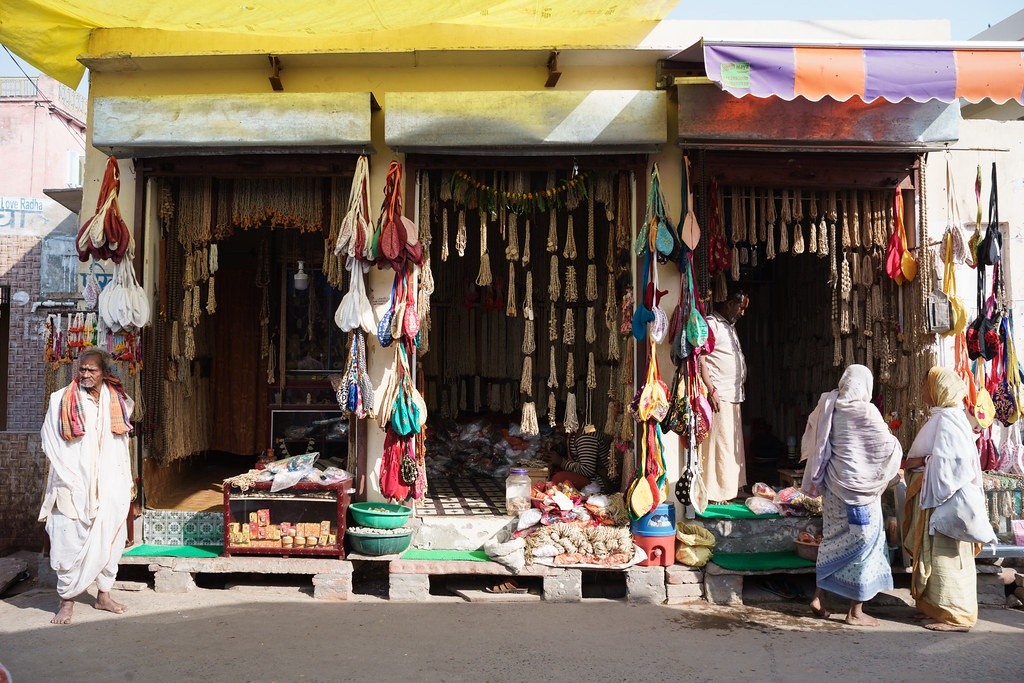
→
[349,502,413,529]
[346,530,412,556]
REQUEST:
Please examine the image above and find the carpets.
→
[121,544,224,557]
[712,550,815,571]
[400,548,491,562]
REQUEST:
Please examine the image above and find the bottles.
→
[505,468,531,515]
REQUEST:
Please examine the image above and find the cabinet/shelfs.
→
[224,478,355,561]
[267,403,348,460]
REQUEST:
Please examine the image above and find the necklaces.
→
[40,313,146,421]
[159,160,346,465]
[441,169,619,434]
[710,176,910,421]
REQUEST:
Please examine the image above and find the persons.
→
[903,365,997,633]
[37,348,136,624]
[695,287,751,504]
[291,340,326,371]
[538,404,616,499]
[796,363,904,627]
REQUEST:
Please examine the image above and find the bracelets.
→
[401,454,419,484]
[708,386,717,396]
[922,455,930,467]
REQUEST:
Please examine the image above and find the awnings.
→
[665,36,1024,110]
